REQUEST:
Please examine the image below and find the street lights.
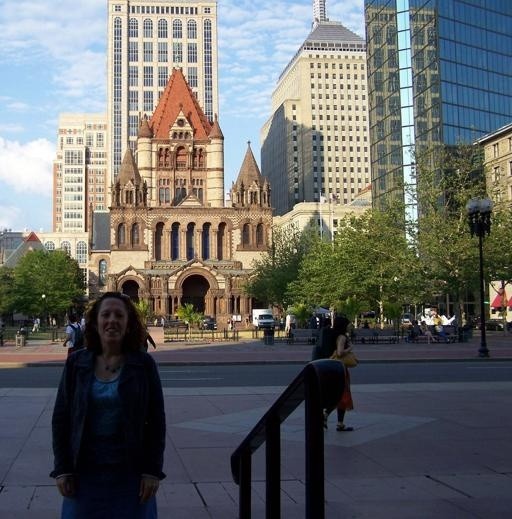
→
[460,193,499,360]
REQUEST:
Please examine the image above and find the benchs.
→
[408,325,458,342]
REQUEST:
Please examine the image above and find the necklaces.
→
[96,355,121,371]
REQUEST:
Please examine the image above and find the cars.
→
[477,319,506,331]
[399,314,414,325]
[199,315,218,332]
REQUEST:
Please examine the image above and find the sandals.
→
[322,414,327,429]
[337,424,353,431]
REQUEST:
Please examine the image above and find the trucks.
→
[250,308,276,330]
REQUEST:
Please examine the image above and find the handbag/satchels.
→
[330,349,359,366]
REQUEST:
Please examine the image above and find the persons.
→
[227,316,236,330]
[323,316,354,431]
[311,312,332,331]
[406,320,450,343]
[161,316,164,327]
[32,317,41,332]
[49,292,166,519]
[143,324,156,352]
[63,314,85,357]
[153,318,158,326]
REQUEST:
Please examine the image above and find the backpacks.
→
[68,324,83,346]
